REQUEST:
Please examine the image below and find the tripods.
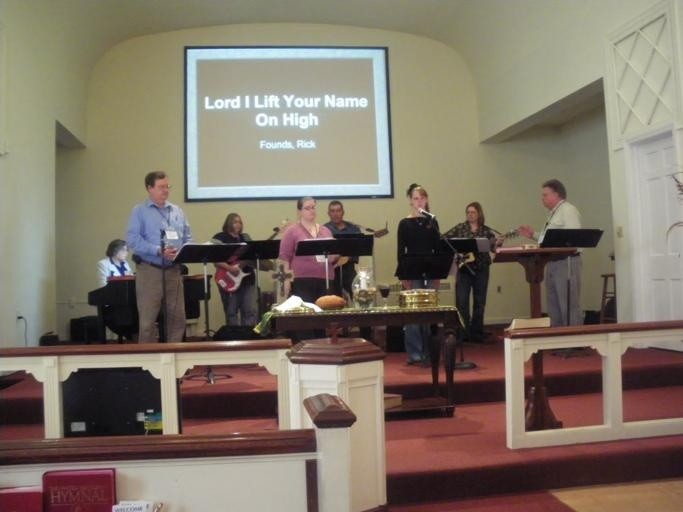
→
[182,262,232,384]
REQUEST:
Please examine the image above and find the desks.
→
[270,306,461,424]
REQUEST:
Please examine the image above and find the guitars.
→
[334,227,387,268]
[215,228,280,293]
[457,230,519,268]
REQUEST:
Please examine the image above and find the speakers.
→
[71,316,106,344]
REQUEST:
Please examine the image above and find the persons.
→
[323,200,361,300]
[211,213,259,327]
[520,178,583,358]
[279,196,340,341]
[441,202,504,349]
[126,171,193,343]
[95,239,133,340]
[394,183,440,363]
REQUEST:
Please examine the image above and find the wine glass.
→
[379,286,390,309]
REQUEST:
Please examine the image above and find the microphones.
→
[418,208,436,220]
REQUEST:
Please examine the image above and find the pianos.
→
[88,275,212,336]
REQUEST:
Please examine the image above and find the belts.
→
[571,252,579,257]
[141,260,176,269]
